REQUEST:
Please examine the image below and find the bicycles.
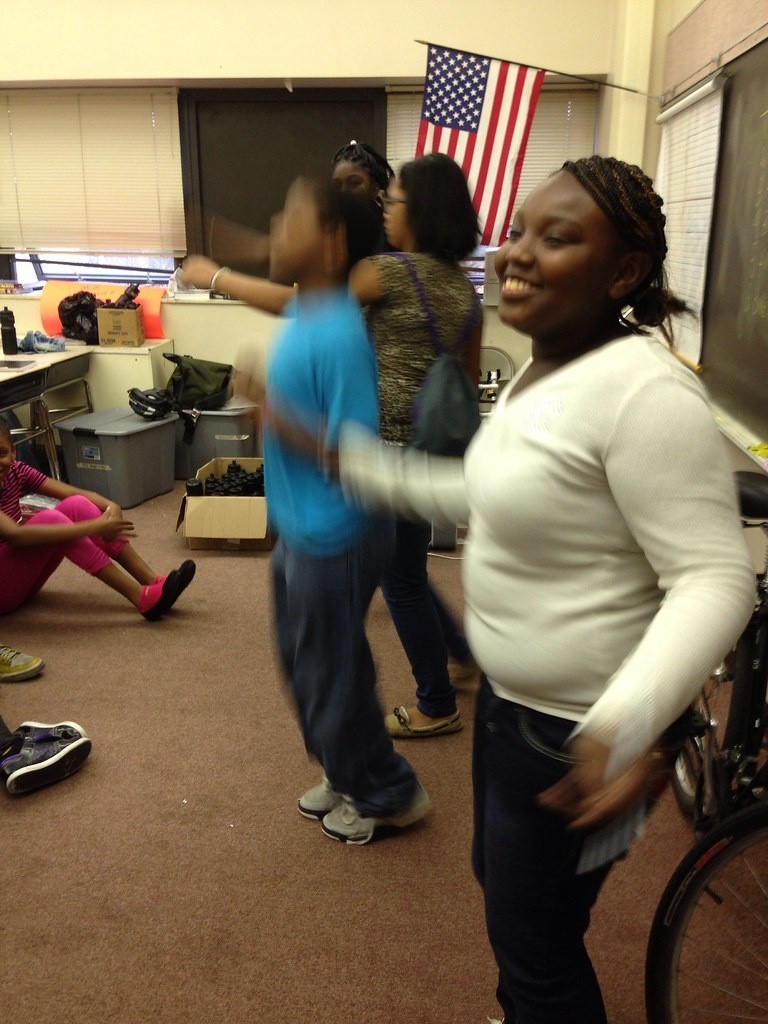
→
[644,470,768,1024]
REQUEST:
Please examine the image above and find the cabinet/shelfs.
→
[0,339,175,445]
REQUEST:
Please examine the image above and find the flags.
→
[416,42,546,248]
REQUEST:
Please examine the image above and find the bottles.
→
[0,307,18,354]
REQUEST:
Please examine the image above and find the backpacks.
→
[384,251,483,457]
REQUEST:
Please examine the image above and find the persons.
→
[0,716,92,795]
[0,415,196,621]
[231,155,759,1024]
[177,144,486,845]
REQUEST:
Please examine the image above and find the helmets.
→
[127,385,177,420]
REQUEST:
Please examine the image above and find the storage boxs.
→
[176,457,274,551]
[175,407,256,478]
[96,305,146,347]
[54,407,181,509]
[485,251,500,306]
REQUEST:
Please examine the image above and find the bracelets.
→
[210,267,231,295]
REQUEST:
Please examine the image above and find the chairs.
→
[430,346,515,550]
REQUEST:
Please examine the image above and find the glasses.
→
[375,189,408,208]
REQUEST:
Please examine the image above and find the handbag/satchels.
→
[58,291,106,344]
[161,352,235,446]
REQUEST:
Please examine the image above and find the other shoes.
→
[383,705,464,740]
[139,559,196,622]
[445,659,471,683]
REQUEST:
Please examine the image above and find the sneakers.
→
[11,720,88,746]
[296,776,346,821]
[0,735,91,795]
[320,780,432,845]
[0,643,45,683]
